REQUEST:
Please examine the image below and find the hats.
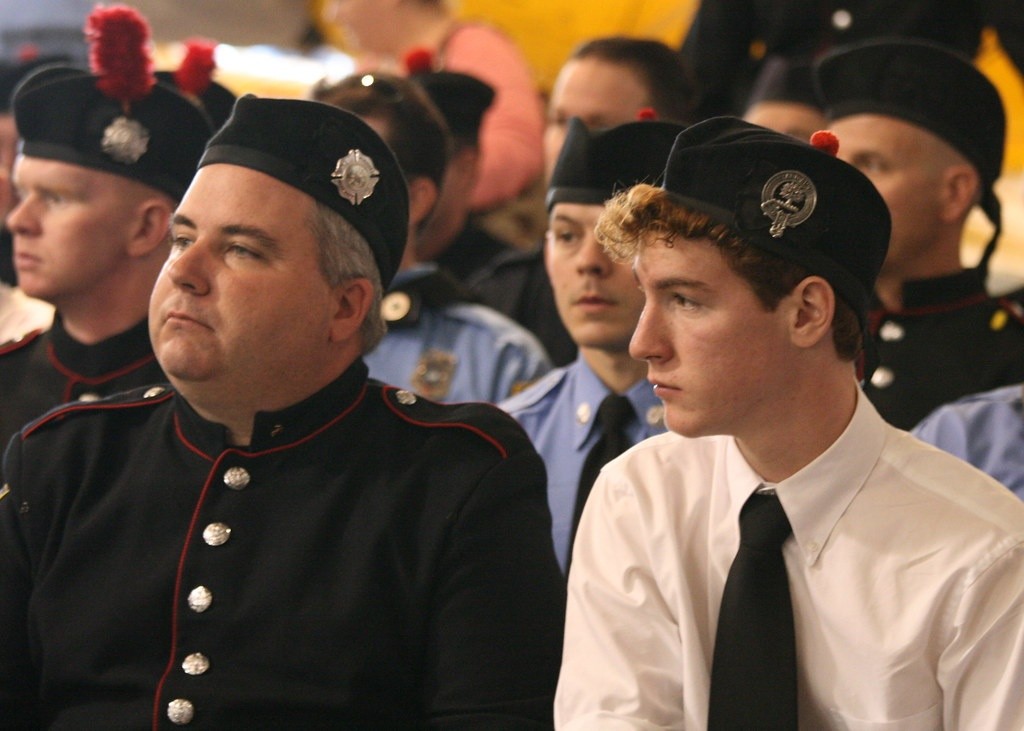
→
[148,72,238,132]
[814,41,1006,188]
[14,3,214,201]
[401,49,494,135]
[663,116,893,332]
[747,51,817,108]
[542,107,685,212]
[196,95,409,296]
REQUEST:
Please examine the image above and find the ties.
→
[707,493,799,731]
[564,392,639,582]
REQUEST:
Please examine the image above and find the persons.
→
[2,0,1024,573]
[0,93,567,731]
[545,118,1024,731]
